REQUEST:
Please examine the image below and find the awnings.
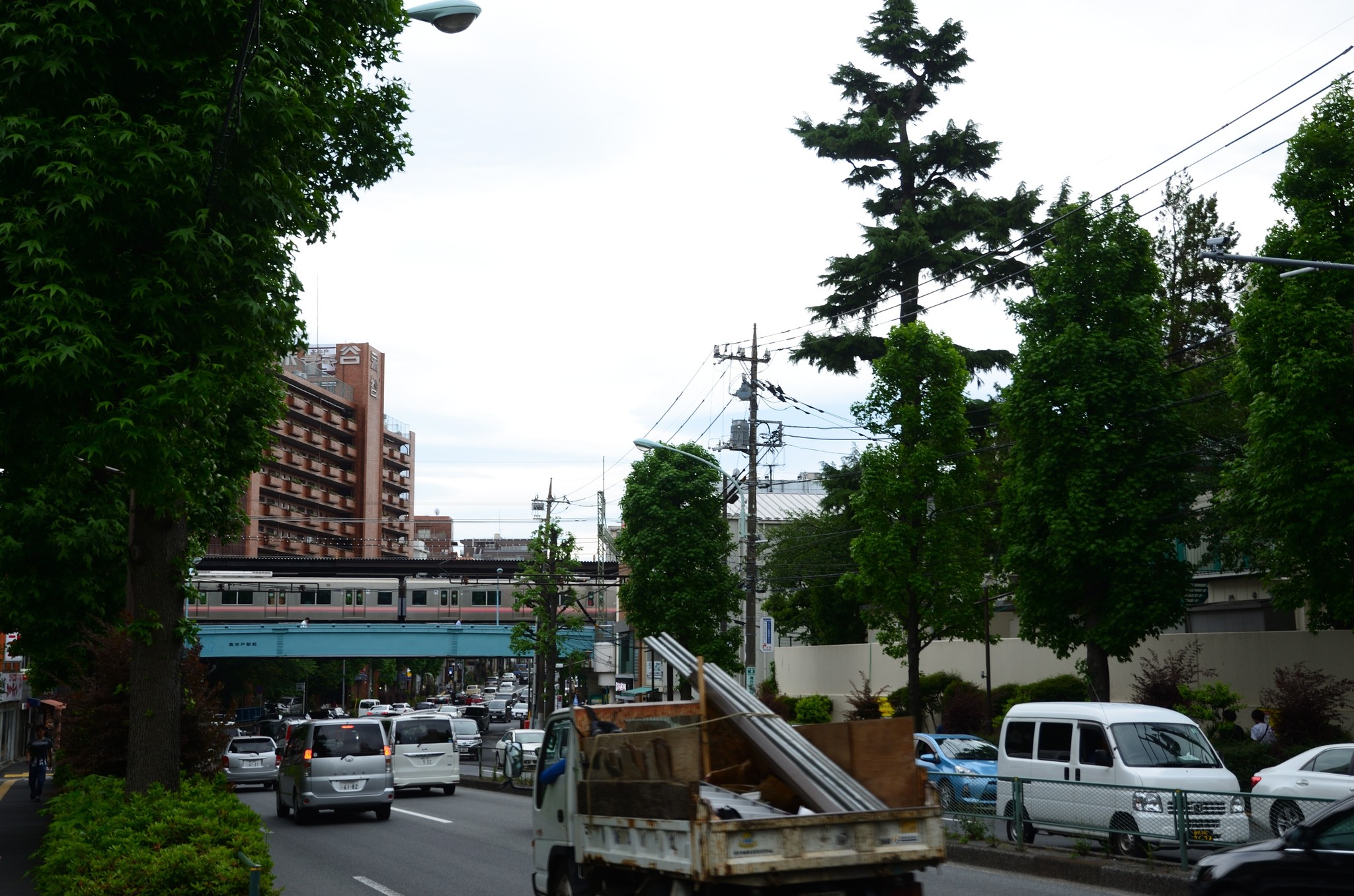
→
[620,687,658,701]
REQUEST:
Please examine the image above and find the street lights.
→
[496,568,503,626]
[631,439,758,694]
[184,556,202,622]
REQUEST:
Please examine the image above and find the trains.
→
[185,569,617,623]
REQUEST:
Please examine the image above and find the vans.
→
[996,700,1249,860]
[380,715,462,796]
[275,717,396,827]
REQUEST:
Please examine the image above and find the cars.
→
[453,717,483,762]
[358,672,532,733]
[1247,743,1354,838]
[494,729,556,769]
[221,736,283,791]
[913,733,999,810]
[1189,794,1354,895]
[211,696,350,739]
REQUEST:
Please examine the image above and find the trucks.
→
[503,697,951,896]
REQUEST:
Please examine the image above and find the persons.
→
[25,718,61,802]
[1209,707,1278,748]
[300,618,310,628]
[540,758,566,789]
[456,617,463,626]
[439,674,459,694]
